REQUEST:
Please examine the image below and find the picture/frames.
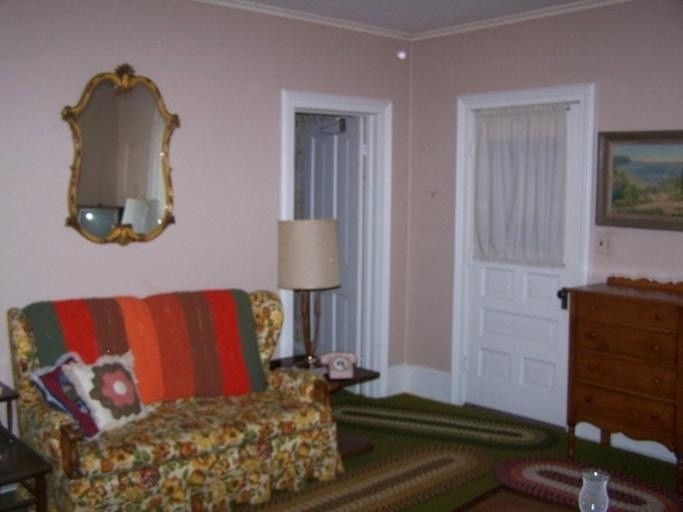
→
[268,352,380,459]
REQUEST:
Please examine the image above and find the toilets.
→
[320,352,357,379]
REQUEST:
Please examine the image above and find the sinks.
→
[6,288,344,512]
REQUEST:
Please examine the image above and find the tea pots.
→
[58,63,180,246]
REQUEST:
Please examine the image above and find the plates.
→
[564,275,683,493]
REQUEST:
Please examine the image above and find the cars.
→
[578,467,611,512]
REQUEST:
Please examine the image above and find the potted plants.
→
[593,129,682,233]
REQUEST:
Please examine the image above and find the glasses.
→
[277,219,342,369]
[119,197,161,235]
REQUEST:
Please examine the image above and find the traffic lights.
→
[27,347,149,442]
